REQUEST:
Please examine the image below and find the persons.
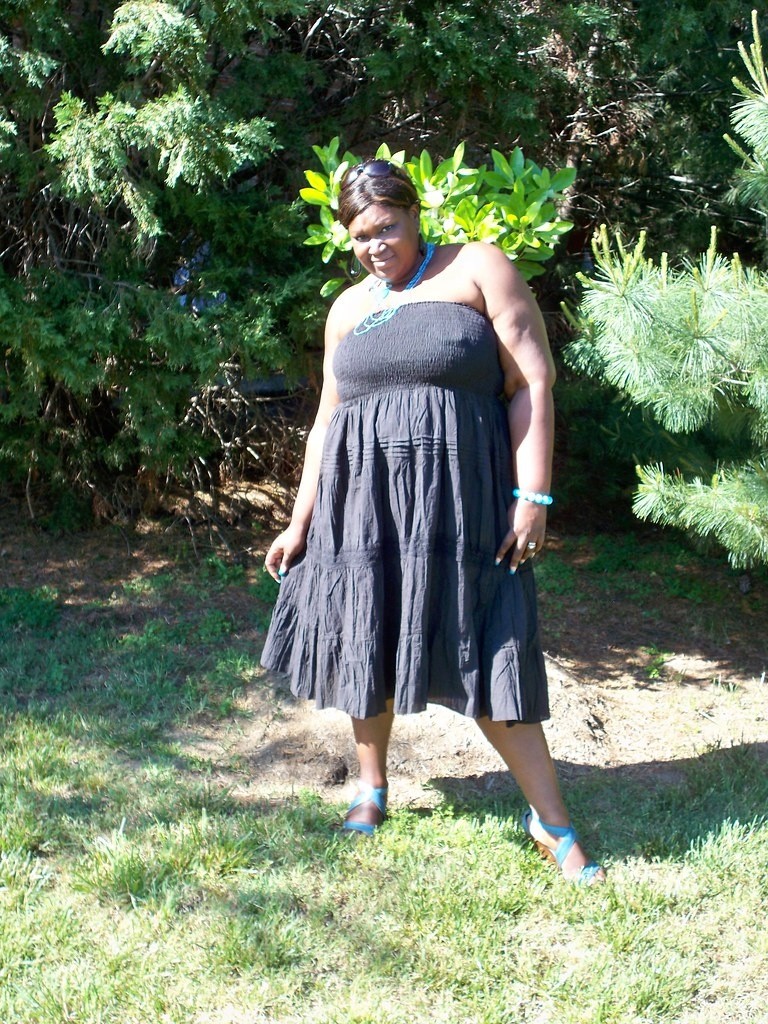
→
[261,158,608,879]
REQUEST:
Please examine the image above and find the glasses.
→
[337,159,407,191]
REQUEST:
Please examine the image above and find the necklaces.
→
[353,243,435,336]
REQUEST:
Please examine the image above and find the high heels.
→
[342,782,389,840]
[521,806,605,894]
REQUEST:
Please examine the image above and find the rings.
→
[527,542,537,549]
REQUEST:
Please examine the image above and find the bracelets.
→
[512,488,554,505]
